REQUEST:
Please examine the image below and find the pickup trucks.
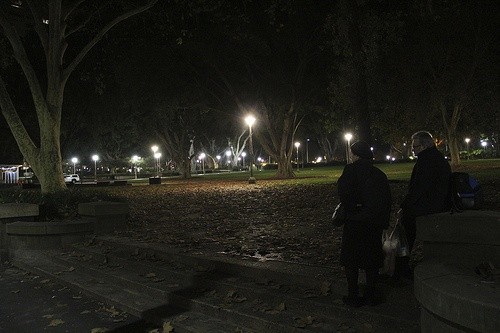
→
[64,174,79,184]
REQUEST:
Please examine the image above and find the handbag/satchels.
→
[330,202,344,227]
[379,233,396,277]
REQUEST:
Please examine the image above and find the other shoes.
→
[359,294,376,306]
[378,270,400,280]
[343,295,359,307]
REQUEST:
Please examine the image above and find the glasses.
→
[413,145,421,148]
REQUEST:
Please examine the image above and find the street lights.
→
[92,154,99,181]
[294,142,300,173]
[464,138,470,160]
[244,113,257,184]
[151,145,162,178]
[71,157,78,174]
[344,132,353,165]
[131,155,138,180]
[200,152,206,174]
[481,141,487,152]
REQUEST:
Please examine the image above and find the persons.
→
[337,140,392,309]
[386,130,452,280]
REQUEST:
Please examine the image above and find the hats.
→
[350,142,373,159]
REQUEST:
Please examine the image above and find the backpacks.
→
[450,171,483,210]
